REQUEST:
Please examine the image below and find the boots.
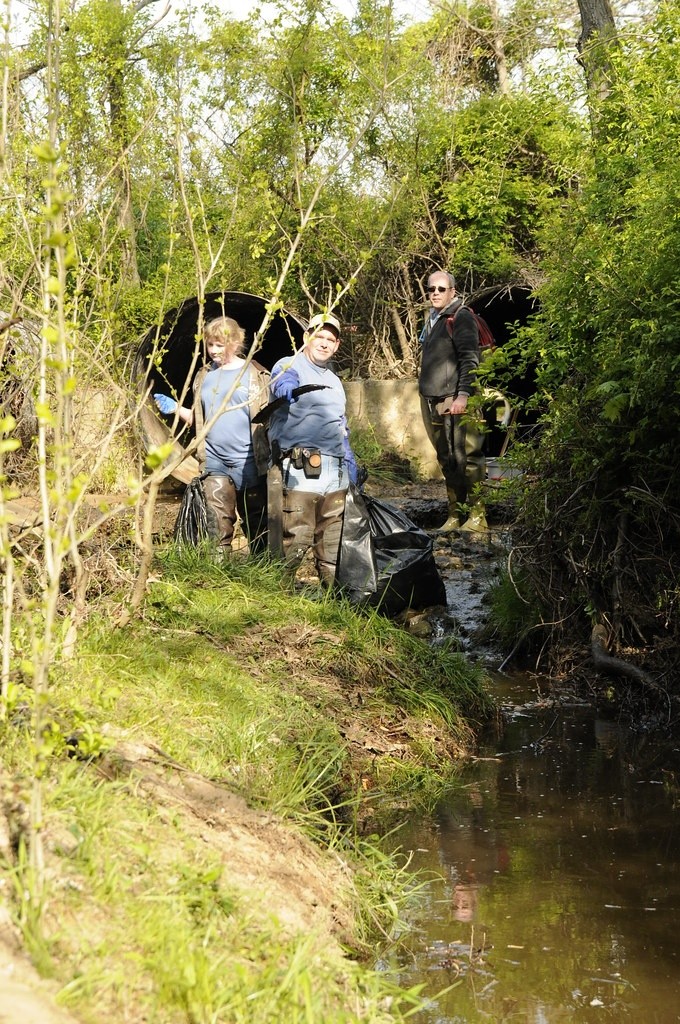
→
[282,487,352,604]
[202,474,270,567]
[431,486,490,534]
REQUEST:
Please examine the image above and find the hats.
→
[308,314,341,339]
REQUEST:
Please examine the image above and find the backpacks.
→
[446,304,497,363]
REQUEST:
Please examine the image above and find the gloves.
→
[272,365,301,404]
[153,393,178,415]
[337,416,363,492]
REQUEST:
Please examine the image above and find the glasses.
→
[427,285,453,293]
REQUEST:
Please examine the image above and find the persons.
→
[154,317,271,563]
[420,271,489,532]
[269,314,364,594]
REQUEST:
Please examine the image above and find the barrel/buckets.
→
[487,463,523,483]
